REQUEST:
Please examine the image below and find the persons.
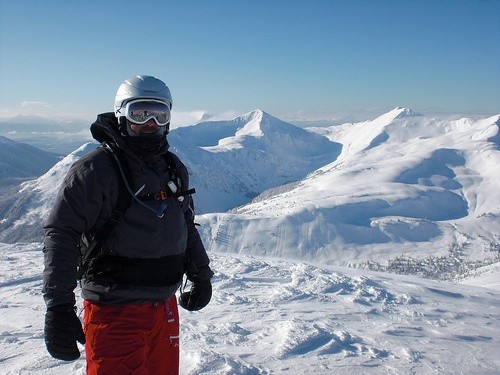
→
[42,73,214,375]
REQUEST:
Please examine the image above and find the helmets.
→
[115,75,173,117]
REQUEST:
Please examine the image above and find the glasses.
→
[124,100,171,127]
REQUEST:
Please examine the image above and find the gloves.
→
[44,309,86,361]
[179,278,212,311]
